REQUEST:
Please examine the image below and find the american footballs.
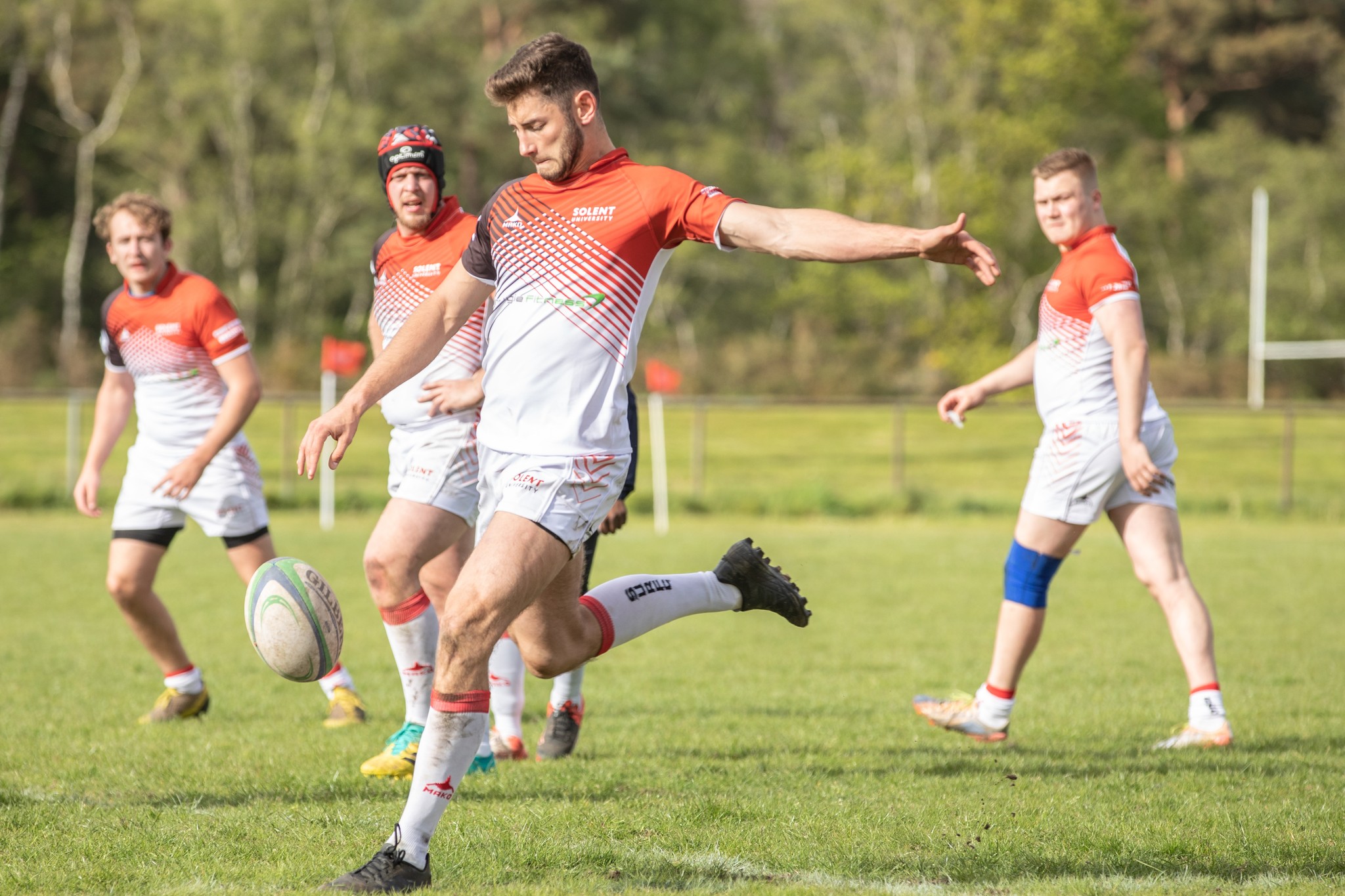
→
[244,556,342,682]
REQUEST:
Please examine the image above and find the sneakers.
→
[488,726,527,760]
[136,680,209,721]
[462,752,496,778]
[911,690,1010,743]
[1151,720,1232,750]
[535,692,585,762]
[321,685,370,728]
[315,823,432,896]
[711,536,812,628]
[360,721,425,780]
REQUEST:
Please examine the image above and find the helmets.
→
[378,124,444,218]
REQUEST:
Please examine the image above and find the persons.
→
[488,377,638,761]
[358,124,499,788]
[295,35,999,896]
[915,151,1234,747]
[72,193,365,732]
